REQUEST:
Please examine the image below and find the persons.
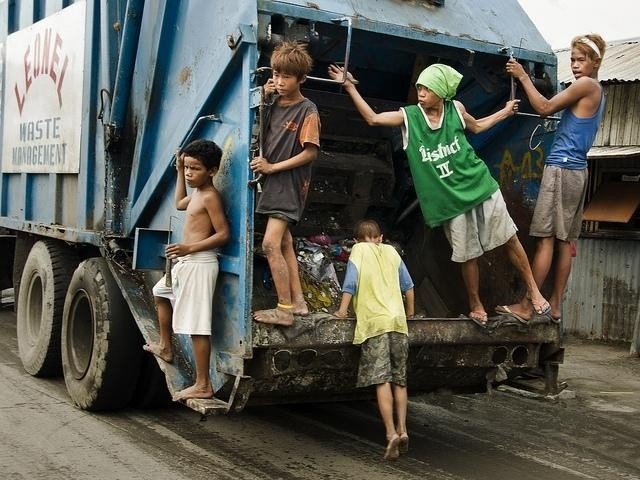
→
[142,138,230,402]
[334,219,416,462]
[494,33,606,323]
[327,63,551,329]
[249,41,322,326]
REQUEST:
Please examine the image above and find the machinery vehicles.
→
[0,0,567,415]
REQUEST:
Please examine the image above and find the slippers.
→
[531,298,552,317]
[469,309,488,329]
[494,303,529,325]
[544,310,562,325]
